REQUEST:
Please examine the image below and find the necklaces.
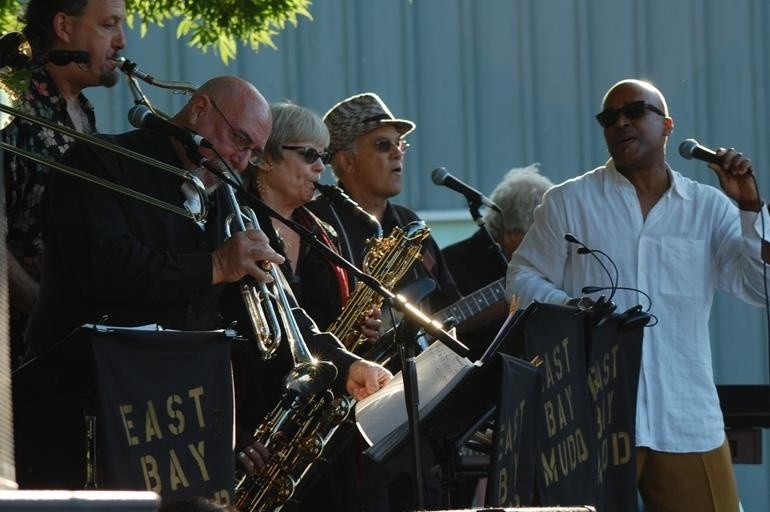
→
[273,226,292,253]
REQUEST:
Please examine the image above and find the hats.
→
[322,93,417,164]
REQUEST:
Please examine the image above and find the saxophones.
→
[110,55,199,95]
[235,180,431,510]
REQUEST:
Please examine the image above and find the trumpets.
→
[220,175,337,391]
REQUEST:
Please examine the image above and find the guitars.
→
[359,278,507,379]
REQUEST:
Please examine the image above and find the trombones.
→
[0,106,207,220]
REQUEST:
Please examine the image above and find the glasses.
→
[211,102,263,167]
[595,100,665,127]
[282,145,333,166]
[343,137,409,155]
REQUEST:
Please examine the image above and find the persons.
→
[439,165,554,357]
[2,1,127,364]
[243,102,382,512]
[315,93,507,511]
[506,79,770,511]
[18,76,392,488]
[160,498,226,512]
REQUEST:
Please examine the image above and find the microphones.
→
[679,139,752,175]
[128,105,213,149]
[431,167,501,213]
[577,248,618,303]
[565,234,614,301]
[581,286,651,312]
